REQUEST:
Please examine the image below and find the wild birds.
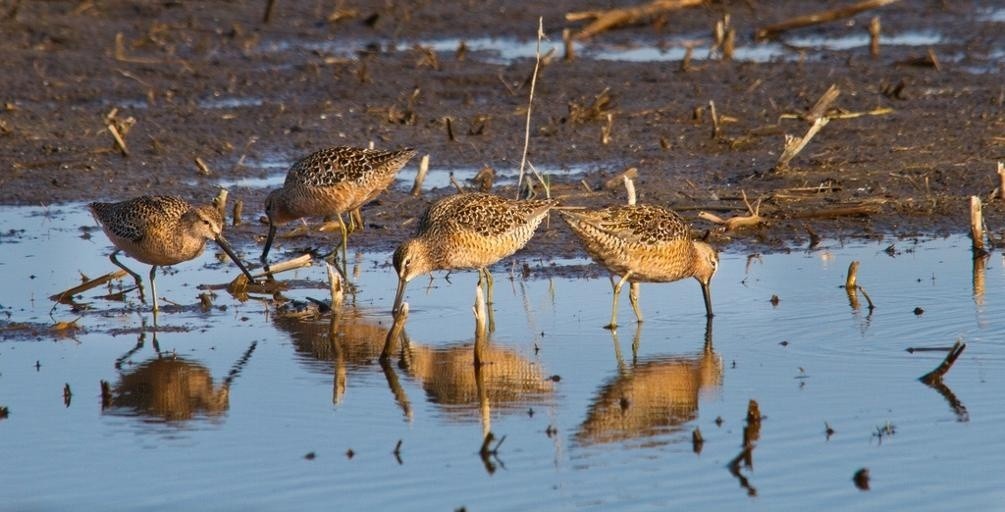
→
[270,288,403,374]
[568,317,723,449]
[399,303,562,412]
[82,193,255,312]
[101,313,258,429]
[259,144,417,264]
[389,196,559,316]
[559,200,720,329]
